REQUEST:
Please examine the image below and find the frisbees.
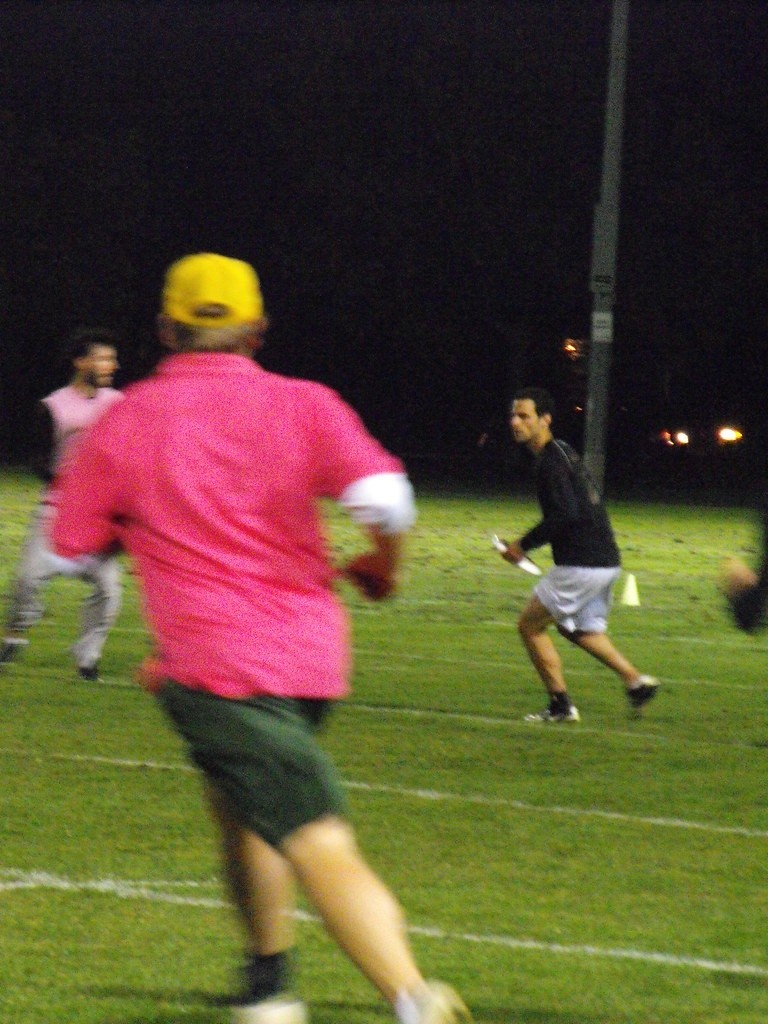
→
[490,534,543,578]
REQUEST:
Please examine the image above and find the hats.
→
[161,253,264,330]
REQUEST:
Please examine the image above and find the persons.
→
[499,389,658,722]
[718,524,768,635]
[52,253,471,1024]
[0,334,130,680]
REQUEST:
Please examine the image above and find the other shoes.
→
[1,638,27,663]
[624,676,661,706]
[77,667,109,686]
[523,704,581,725]
[207,979,311,1024]
